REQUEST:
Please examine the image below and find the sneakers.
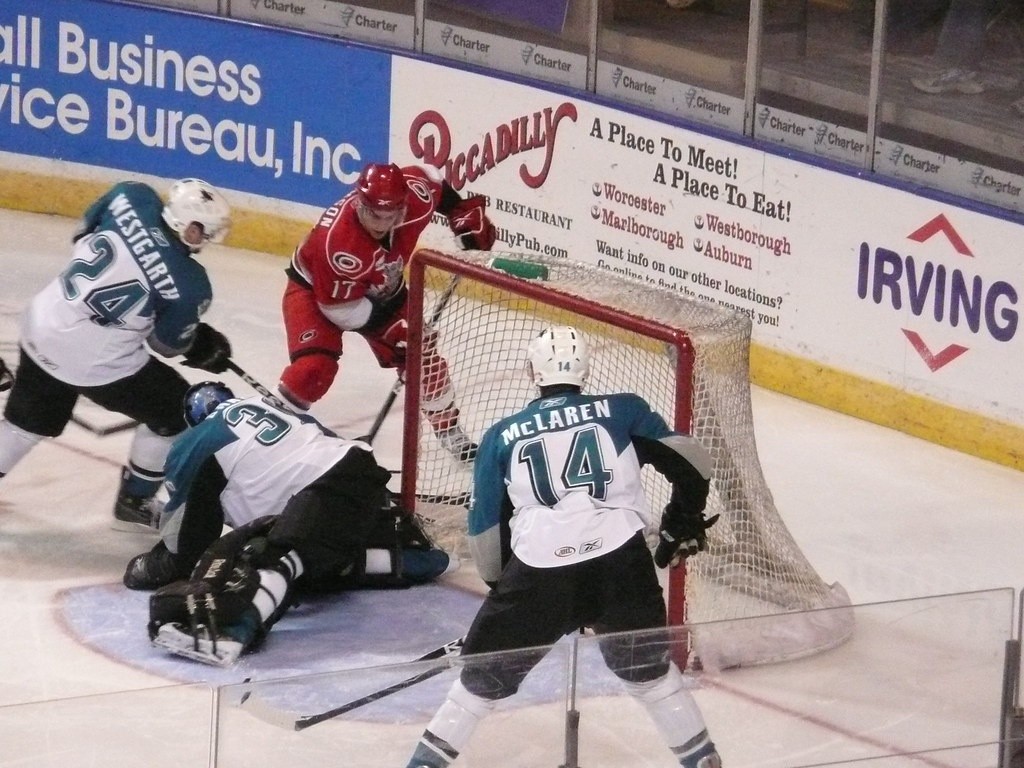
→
[153,622,253,666]
[110,490,166,535]
[437,429,479,462]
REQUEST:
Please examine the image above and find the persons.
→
[408,326,724,768]
[269,161,497,467]
[123,381,450,667]
[0,177,231,534]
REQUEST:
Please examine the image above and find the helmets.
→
[161,178,233,254]
[357,162,411,230]
[525,327,590,387]
[183,380,233,428]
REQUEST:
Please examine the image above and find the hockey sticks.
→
[351,273,460,446]
[3,371,145,438]
[391,490,472,512]
[229,359,295,417]
[239,513,720,731]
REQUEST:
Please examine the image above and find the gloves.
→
[654,504,706,568]
[377,317,411,355]
[181,324,231,374]
[446,195,495,252]
